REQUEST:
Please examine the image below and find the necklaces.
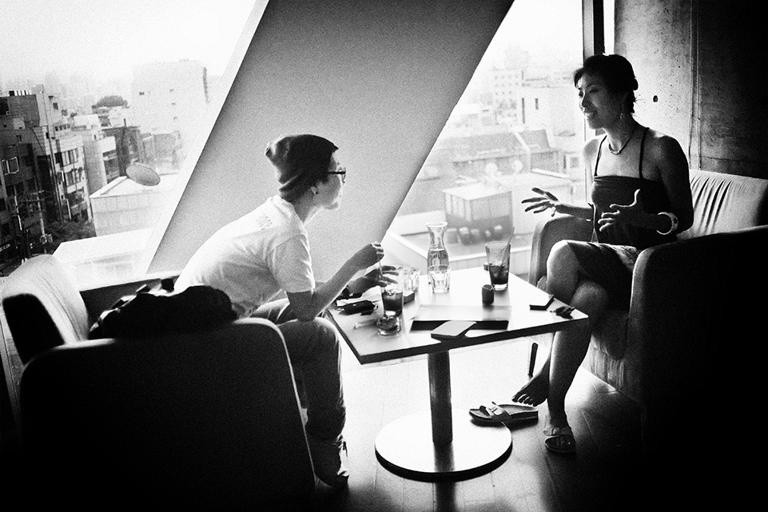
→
[608,125,641,155]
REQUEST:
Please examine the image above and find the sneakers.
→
[306,433,351,488]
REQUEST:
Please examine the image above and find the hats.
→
[263,134,339,202]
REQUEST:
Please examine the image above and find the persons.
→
[172,134,400,488]
[511,53,694,452]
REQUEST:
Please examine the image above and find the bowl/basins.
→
[397,265,422,294]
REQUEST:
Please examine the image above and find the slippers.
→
[543,414,577,455]
[468,401,539,427]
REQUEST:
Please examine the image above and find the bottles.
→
[425,221,449,282]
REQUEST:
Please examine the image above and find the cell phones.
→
[529,295,554,307]
[344,300,375,315]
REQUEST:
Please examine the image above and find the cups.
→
[428,264,449,294]
[484,239,511,293]
[378,270,403,315]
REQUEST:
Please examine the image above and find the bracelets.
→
[343,286,350,300]
[656,211,679,236]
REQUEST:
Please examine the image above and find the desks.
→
[325,266,592,484]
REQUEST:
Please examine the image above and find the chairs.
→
[0,250,326,510]
[526,168,767,458]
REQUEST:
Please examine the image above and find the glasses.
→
[326,167,347,183]
